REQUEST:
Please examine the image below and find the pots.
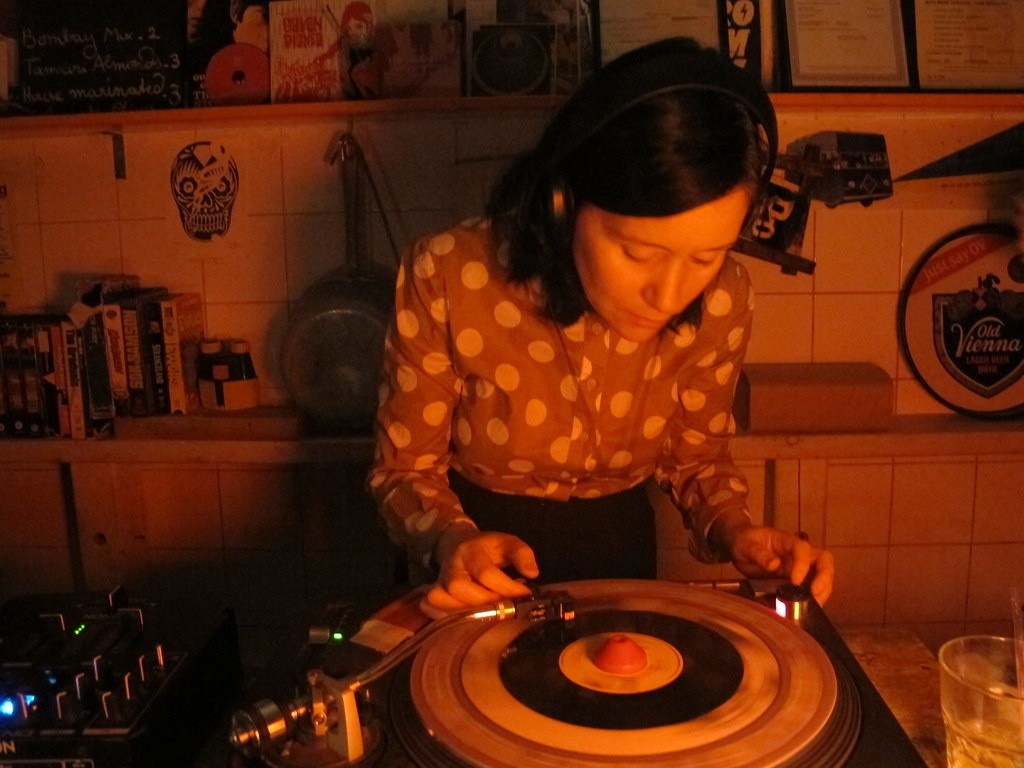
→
[279,131,395,428]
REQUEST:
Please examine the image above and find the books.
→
[0,274,204,440]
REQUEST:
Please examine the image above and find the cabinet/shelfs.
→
[1,462,1024,662]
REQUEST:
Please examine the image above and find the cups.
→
[938,634,1024,768]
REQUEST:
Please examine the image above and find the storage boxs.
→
[737,362,894,435]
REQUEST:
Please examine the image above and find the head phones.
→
[533,52,777,236]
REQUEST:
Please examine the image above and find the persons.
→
[367,35,833,620]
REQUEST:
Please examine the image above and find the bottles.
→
[197,339,255,405]
